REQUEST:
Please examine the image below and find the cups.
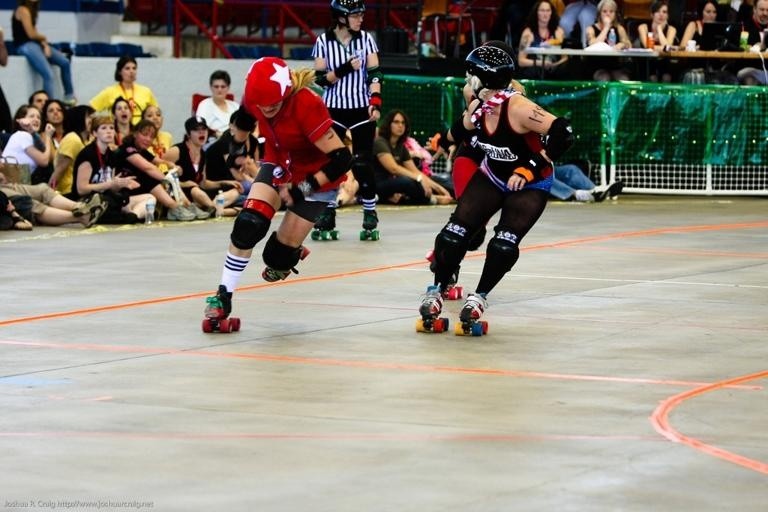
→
[685,40,696,52]
[739,31,750,51]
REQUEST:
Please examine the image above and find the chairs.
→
[627,19,656,47]
[507,22,529,61]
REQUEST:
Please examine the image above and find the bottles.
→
[215,190,225,220]
[144,194,155,225]
[608,28,617,47]
[647,32,655,49]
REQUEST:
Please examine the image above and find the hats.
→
[230,110,255,130]
[185,116,209,128]
[114,56,136,82]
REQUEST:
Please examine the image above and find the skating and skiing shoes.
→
[360,209,380,241]
[426,249,435,272]
[311,207,339,240]
[261,244,310,283]
[454,292,487,336]
[201,284,241,334]
[415,283,448,334]
[439,265,463,300]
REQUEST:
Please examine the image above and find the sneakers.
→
[167,206,195,221]
[595,182,623,198]
[588,190,607,203]
[73,193,100,210]
[186,203,210,220]
[73,201,108,228]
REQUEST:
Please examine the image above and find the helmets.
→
[481,41,519,73]
[466,45,514,91]
[330,0,365,14]
[244,56,296,106]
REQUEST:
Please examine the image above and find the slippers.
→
[3,210,32,231]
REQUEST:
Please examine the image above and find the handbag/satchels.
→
[161,169,181,205]
[1,156,31,186]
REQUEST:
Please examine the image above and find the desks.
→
[524,47,660,81]
[660,50,764,85]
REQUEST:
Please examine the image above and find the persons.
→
[2,1,452,230]
[204,56,352,318]
[447,40,531,209]
[518,0,768,84]
[548,163,625,202]
[419,48,570,320]
